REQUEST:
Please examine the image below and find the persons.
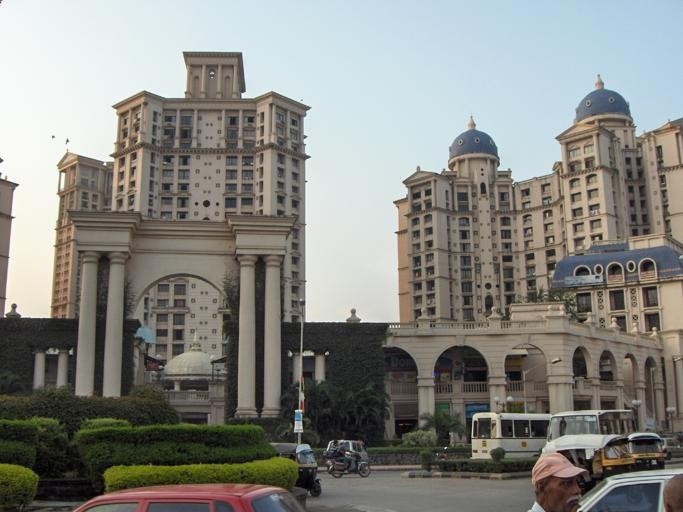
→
[663,472,683,512]
[526,452,588,512]
[323,439,364,472]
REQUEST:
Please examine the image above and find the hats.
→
[531,452,589,485]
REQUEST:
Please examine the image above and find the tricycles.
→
[268,441,322,497]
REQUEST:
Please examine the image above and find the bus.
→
[470,412,552,459]
[546,409,636,446]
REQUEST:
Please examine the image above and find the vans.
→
[545,434,635,482]
[324,440,369,466]
[614,431,666,472]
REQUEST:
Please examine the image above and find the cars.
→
[70,482,308,511]
[571,467,683,511]
[660,437,682,460]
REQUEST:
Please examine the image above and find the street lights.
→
[296,296,306,445]
[522,357,561,411]
[493,396,512,412]
[210,354,216,383]
[666,406,675,433]
[632,399,641,430]
[215,367,221,382]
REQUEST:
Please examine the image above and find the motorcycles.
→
[431,442,450,470]
[321,449,371,479]
